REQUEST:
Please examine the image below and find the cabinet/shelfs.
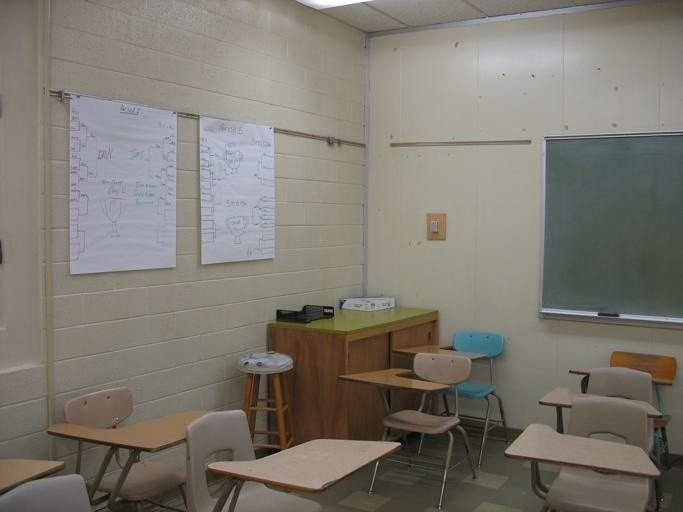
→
[267,306,440,448]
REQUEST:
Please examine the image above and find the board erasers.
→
[598,313,619,317]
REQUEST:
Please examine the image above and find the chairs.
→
[565,351,677,455]
[338,351,477,511]
[181,410,400,512]
[504,397,664,512]
[1,457,90,511]
[537,366,668,445]
[398,330,508,470]
[47,387,205,511]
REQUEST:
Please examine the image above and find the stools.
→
[239,349,293,451]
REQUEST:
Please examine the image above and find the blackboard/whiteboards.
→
[537,130,683,323]
